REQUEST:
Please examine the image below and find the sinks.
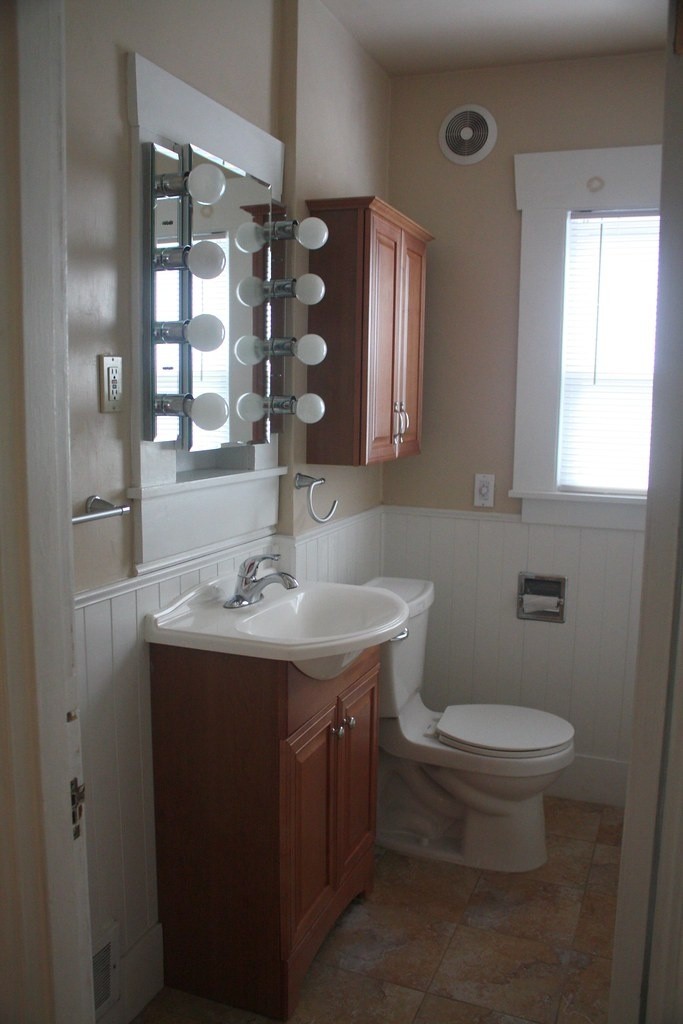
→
[144,568,409,681]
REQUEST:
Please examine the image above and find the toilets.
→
[360,575,576,874]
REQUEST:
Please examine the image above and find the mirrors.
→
[180,141,270,454]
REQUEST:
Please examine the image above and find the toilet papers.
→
[522,594,560,614]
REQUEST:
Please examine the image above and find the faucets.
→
[223,553,300,609]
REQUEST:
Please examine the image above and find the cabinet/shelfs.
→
[151,663,378,1024]
[304,196,436,466]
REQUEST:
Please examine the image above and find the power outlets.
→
[98,353,123,414]
